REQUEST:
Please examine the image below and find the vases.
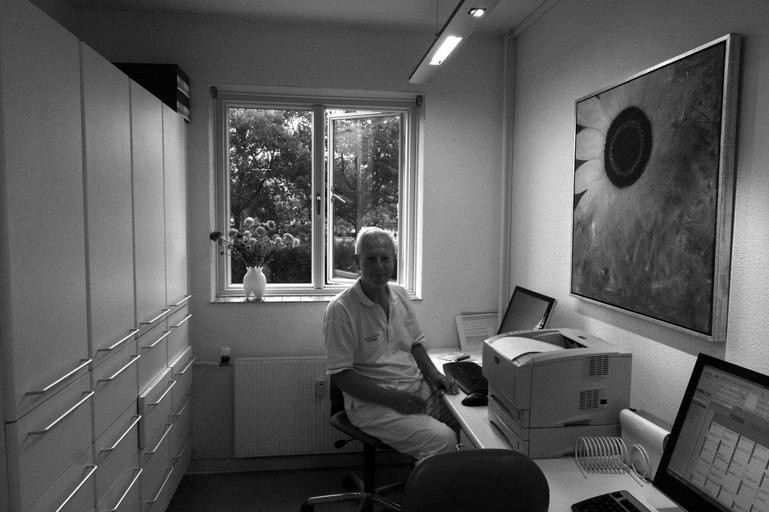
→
[243,266,267,301]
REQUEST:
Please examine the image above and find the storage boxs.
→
[112,62,192,124]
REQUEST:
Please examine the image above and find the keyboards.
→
[572,490,651,512]
[443,362,487,395]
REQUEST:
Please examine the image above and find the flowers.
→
[210,216,301,268]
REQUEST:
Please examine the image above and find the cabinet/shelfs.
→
[0,1,196,512]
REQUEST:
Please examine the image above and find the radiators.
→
[232,355,383,458]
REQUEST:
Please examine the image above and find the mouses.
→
[462,392,488,406]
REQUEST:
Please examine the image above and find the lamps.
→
[408,0,503,86]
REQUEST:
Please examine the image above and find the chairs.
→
[400,449,549,511]
[300,373,407,512]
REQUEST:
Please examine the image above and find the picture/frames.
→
[570,32,743,343]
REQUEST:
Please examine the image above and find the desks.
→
[425,347,689,512]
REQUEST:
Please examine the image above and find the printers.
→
[483,327,631,458]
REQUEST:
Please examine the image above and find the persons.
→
[320,225,460,468]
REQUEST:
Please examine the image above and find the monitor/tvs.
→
[497,285,557,336]
[653,352,769,511]
